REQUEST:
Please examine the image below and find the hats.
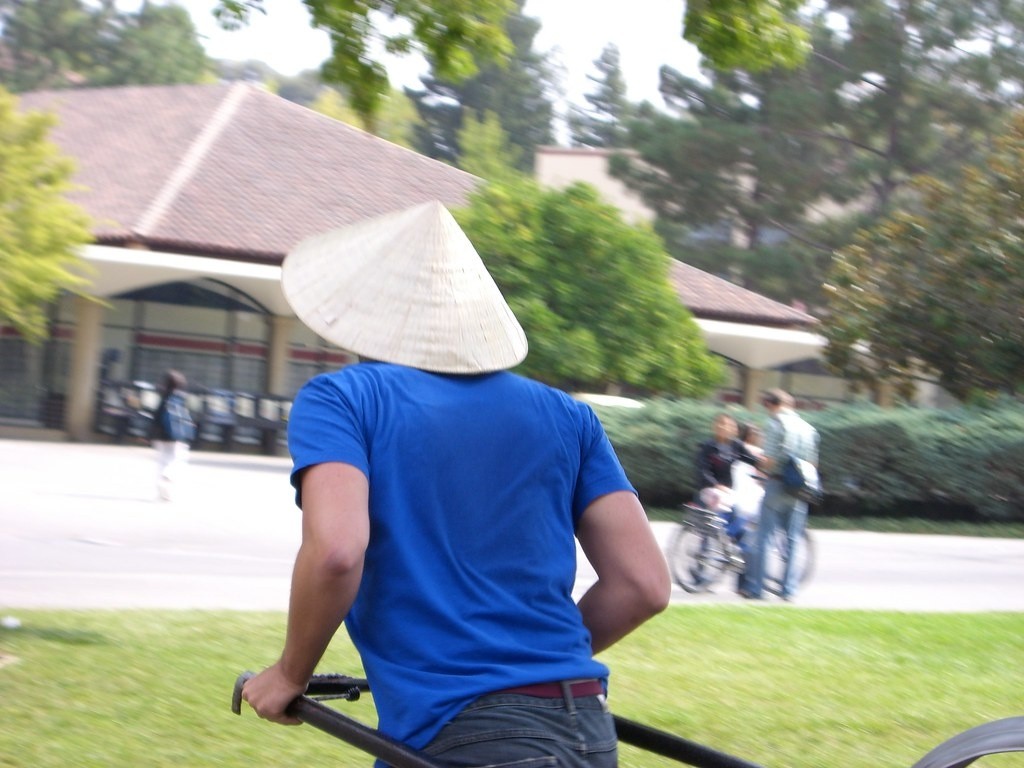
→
[279,196,529,376]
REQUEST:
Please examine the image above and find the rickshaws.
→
[229,668,1024,767]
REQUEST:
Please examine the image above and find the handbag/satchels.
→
[785,456,823,505]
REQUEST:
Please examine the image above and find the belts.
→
[487,679,604,699]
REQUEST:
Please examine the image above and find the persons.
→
[695,386,821,604]
[142,368,193,500]
[243,205,671,768]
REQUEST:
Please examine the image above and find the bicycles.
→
[668,500,819,598]
